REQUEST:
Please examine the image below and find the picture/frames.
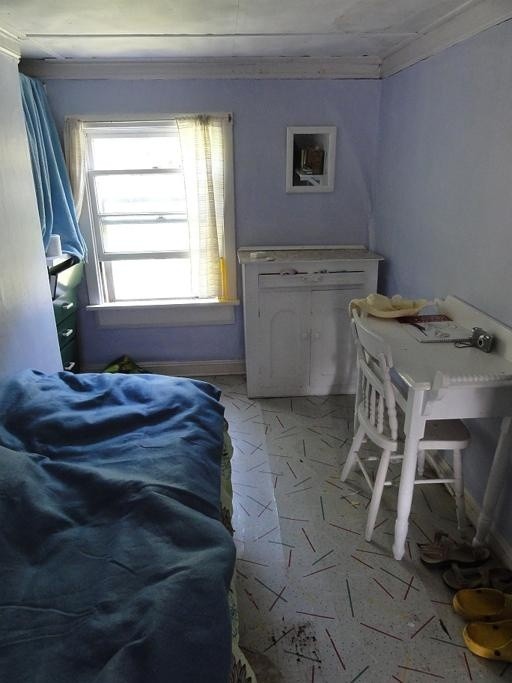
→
[284,126,337,194]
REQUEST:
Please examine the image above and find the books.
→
[404,321,475,344]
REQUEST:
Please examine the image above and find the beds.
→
[0,372,251,683]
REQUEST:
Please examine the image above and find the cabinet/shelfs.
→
[44,241,83,375]
[236,244,384,399]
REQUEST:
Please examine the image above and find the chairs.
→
[342,342,470,542]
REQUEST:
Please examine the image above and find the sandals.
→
[416,529,491,568]
[440,563,512,592]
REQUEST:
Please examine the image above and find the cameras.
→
[471,327,496,353]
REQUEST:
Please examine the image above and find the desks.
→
[353,294,511,550]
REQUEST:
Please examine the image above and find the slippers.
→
[452,588,512,620]
[462,619,512,663]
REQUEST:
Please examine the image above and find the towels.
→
[348,293,427,319]
